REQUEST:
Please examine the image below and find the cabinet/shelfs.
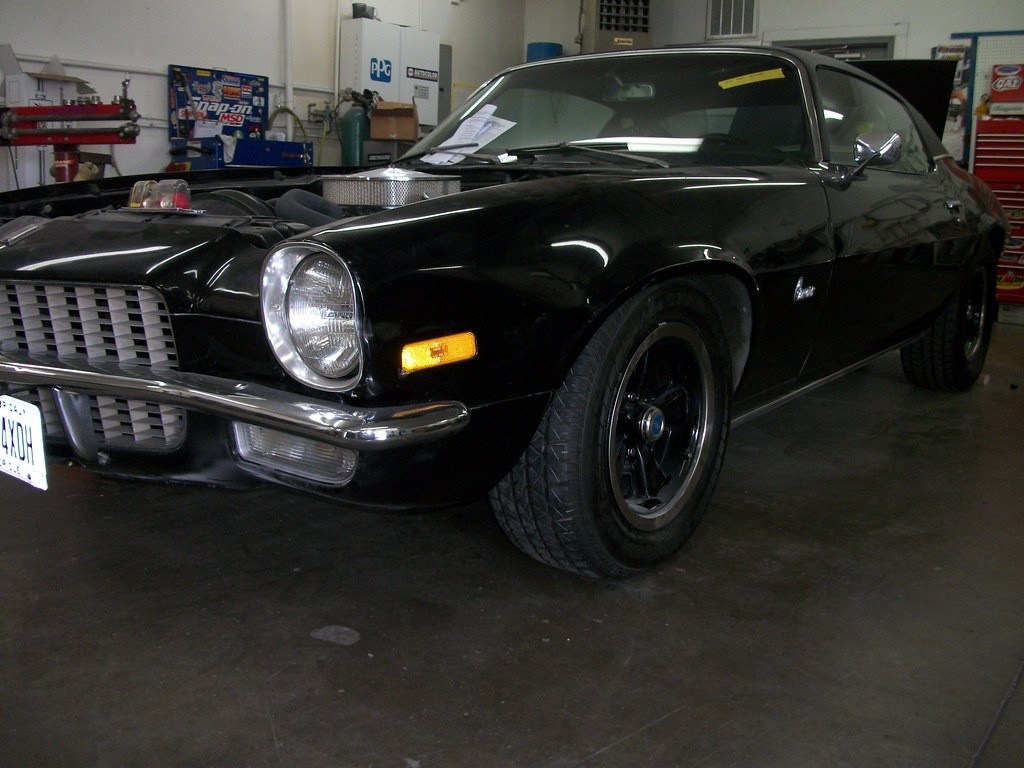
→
[340,18,440,126]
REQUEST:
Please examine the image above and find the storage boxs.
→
[0,43,97,190]
[370,96,419,142]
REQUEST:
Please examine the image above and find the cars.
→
[0,45,1011,584]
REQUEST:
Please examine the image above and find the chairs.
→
[729,83,804,157]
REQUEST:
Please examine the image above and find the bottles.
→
[111,95,122,104]
[91,96,101,105]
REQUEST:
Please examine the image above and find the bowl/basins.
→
[63,99,74,105]
[77,99,89,105]
